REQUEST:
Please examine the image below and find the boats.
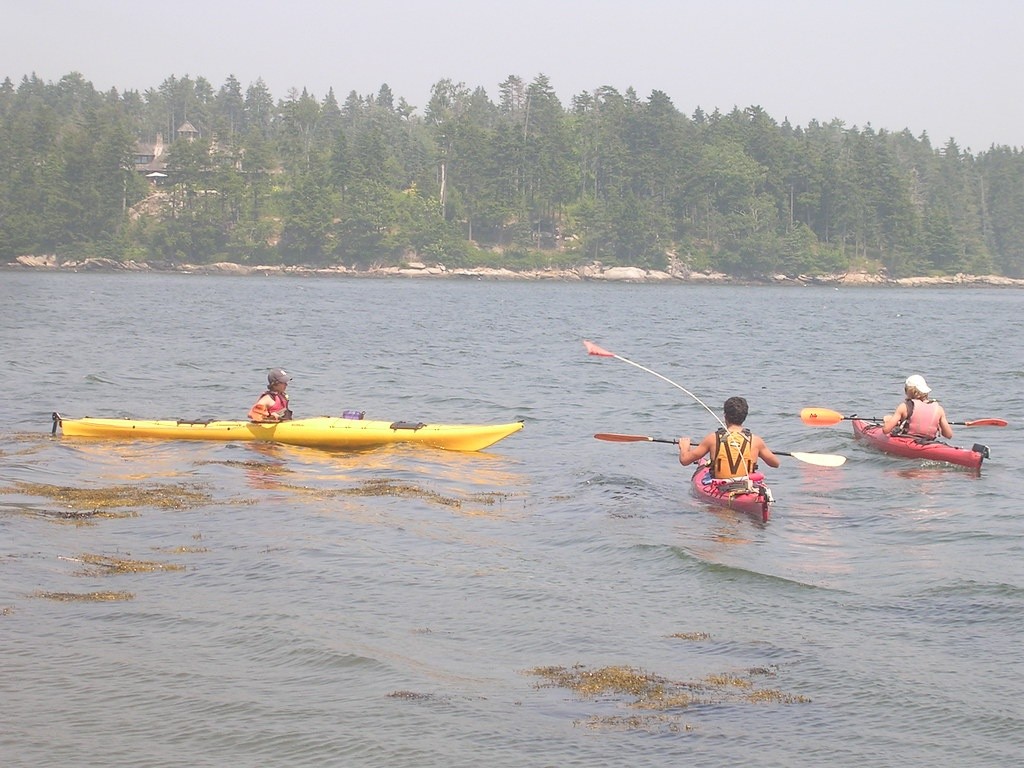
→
[51,410,524,456]
[851,412,990,472]
[690,456,774,527]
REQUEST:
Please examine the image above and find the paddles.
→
[250,417,283,424]
[799,408,1009,430]
[595,432,847,468]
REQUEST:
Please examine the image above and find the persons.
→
[882,375,953,440]
[248,368,292,421]
[678,397,780,479]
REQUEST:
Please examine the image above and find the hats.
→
[267,369,292,389]
[906,375,931,393]
